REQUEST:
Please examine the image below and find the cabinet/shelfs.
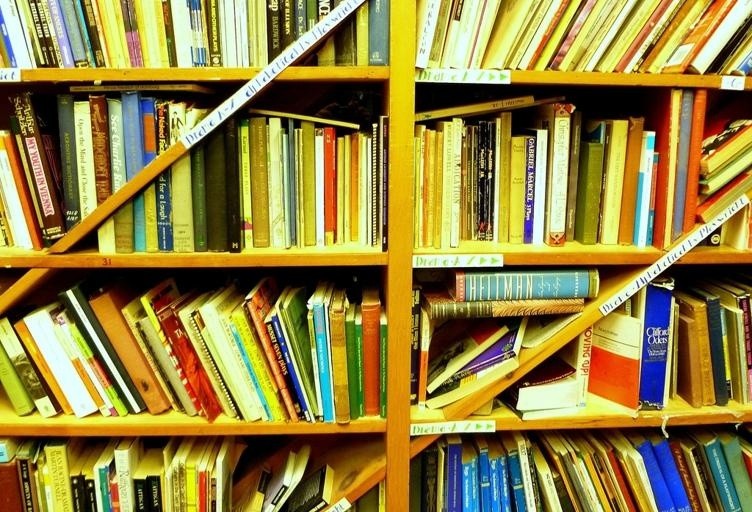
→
[398,0,750,512]
[0,0,397,511]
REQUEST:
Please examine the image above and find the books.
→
[417,87,751,248]
[410,270,752,421]
[415,432,752,512]
[1,0,389,65]
[1,84,388,256]
[2,439,384,510]
[0,277,387,426]
[418,0,751,75]
